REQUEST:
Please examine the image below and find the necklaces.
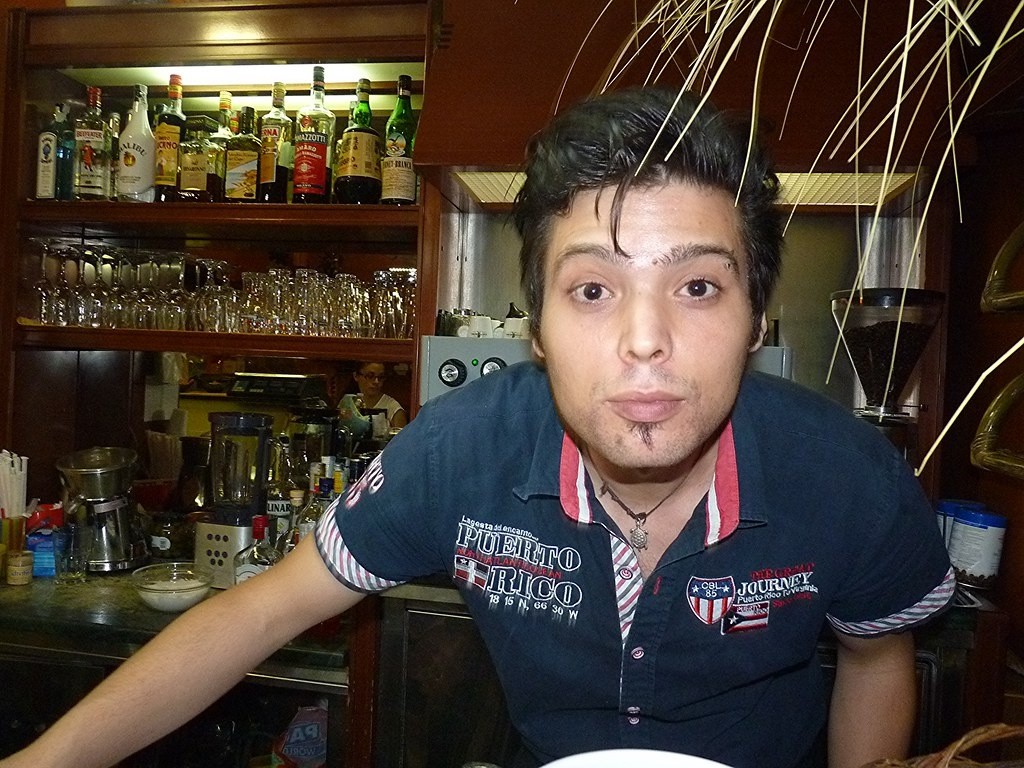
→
[584,441,705,553]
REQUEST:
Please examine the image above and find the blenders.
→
[194,412,274,593]
[829,287,944,457]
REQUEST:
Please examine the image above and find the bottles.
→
[232,435,373,585]
[34,66,417,205]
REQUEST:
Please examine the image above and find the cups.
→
[55,526,93,584]
[230,268,412,339]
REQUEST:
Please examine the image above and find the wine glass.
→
[24,237,228,333]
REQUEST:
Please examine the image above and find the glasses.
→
[357,372,387,383]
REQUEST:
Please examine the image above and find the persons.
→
[1,88,956,768]
[336,361,408,435]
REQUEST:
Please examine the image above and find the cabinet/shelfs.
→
[374,160,1009,768]
[0,0,426,768]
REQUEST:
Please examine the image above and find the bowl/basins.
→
[132,562,215,611]
